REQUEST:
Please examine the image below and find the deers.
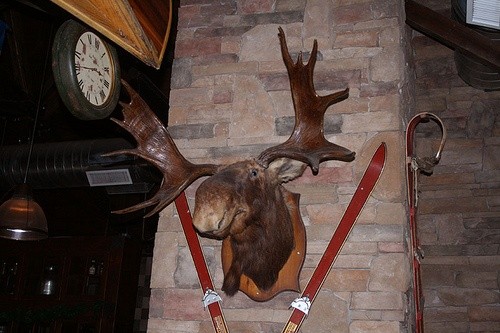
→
[106,24,358,301]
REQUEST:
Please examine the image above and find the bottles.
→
[86,259,97,295]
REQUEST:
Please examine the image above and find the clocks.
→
[51,18,121,121]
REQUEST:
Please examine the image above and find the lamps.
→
[0,42,54,241]
[451,0,500,92]
